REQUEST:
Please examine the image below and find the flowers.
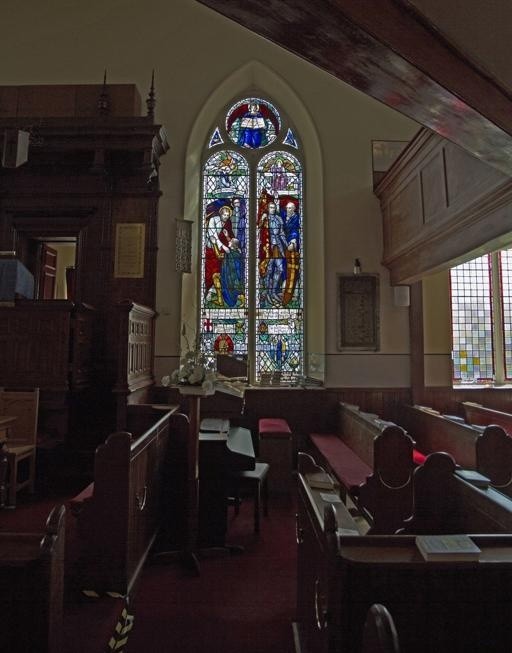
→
[161,324,216,389]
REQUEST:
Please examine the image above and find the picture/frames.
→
[337,274,380,351]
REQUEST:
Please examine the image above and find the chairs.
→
[1,390,295,652]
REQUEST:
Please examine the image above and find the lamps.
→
[353,258,361,274]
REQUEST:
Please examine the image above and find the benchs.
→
[288,389,511,652]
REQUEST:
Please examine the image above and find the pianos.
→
[199,418,255,558]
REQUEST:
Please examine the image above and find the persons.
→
[207,196,247,309]
[259,200,302,308]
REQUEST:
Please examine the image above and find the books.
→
[305,471,333,490]
[415,535,481,562]
[338,401,492,484]
